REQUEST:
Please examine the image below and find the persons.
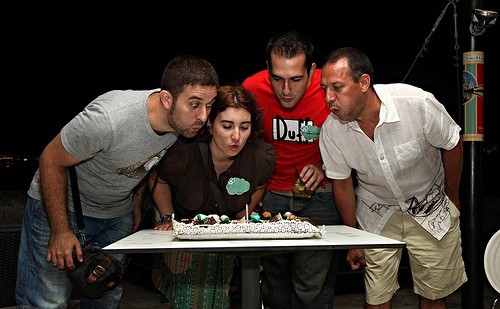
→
[240,34,332,309]
[147,87,275,309]
[14,56,218,309]
[319,49,468,309]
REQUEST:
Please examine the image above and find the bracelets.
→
[161,214,172,222]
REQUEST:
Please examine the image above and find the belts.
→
[267,181,333,197]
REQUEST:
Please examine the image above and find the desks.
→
[100,225,407,309]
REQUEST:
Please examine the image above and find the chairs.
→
[483,230,500,309]
[0,224,21,308]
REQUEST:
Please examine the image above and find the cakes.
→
[183,212,317,227]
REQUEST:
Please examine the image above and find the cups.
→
[293,183,312,211]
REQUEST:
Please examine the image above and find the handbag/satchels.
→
[63,240,124,303]
